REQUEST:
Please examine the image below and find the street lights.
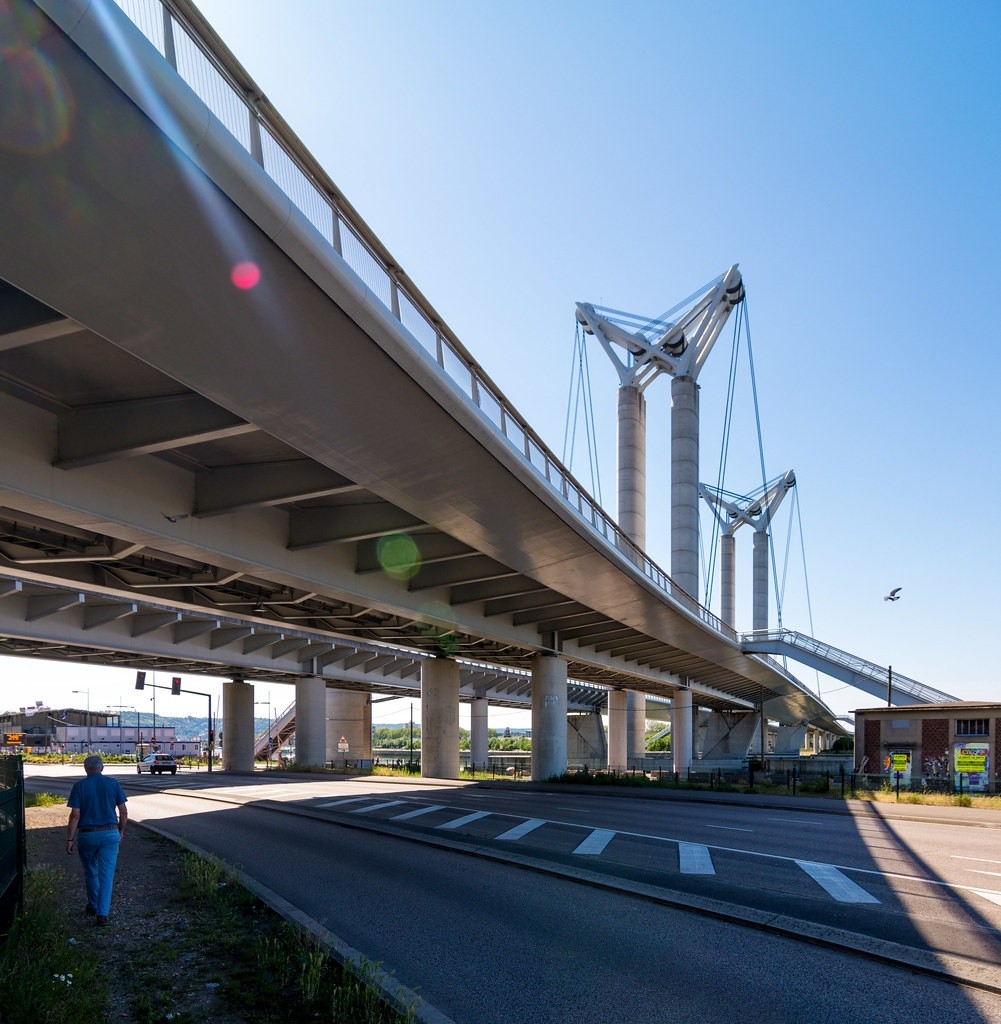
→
[72,690,90,756]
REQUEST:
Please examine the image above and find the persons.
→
[921,752,949,787]
[66,756,128,926]
[278,751,288,767]
[375,756,411,770]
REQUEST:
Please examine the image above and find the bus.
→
[0,733,61,756]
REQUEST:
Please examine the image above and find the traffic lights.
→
[172,677,181,695]
[209,730,213,741]
[135,671,146,689]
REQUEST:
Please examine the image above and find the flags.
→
[60,710,67,720]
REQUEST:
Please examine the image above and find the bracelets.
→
[68,840,73,841]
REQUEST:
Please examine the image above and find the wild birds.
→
[885,587,901,601]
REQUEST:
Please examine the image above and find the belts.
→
[79,825,118,831]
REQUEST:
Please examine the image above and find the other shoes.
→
[97,914,109,922]
[86,903,98,915]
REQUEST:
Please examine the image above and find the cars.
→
[136,753,177,775]
